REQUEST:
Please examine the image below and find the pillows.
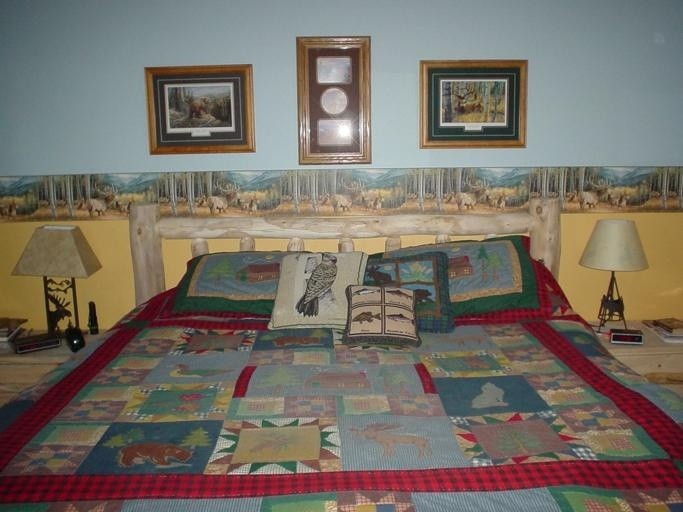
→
[268,251,368,331]
[170,251,313,315]
[342,285,422,347]
[364,236,549,334]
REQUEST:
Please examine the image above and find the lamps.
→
[9,226,102,338]
[579,218,648,334]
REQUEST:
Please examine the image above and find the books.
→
[1,316,28,354]
[642,318,683,343]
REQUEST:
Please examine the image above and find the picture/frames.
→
[296,37,371,163]
[419,61,526,149]
[143,65,255,154]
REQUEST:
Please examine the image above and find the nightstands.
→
[591,313,683,375]
[0,328,103,386]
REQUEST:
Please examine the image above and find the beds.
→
[0,198,683,511]
